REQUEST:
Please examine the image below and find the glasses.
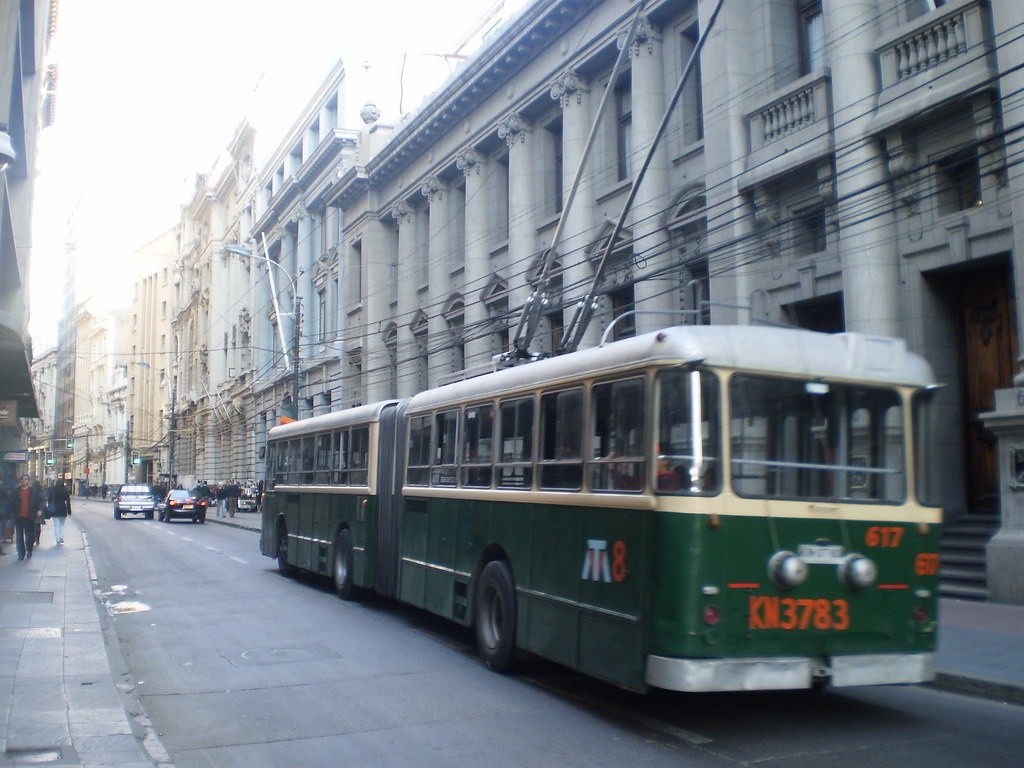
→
[21,477,29,480]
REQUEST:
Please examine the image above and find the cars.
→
[157,489,207,524]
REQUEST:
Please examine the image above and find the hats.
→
[203,480,207,486]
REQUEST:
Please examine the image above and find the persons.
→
[101,478,238,517]
[0,474,72,561]
[92,483,98,498]
[609,442,681,492]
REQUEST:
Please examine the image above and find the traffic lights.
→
[45,451,53,466]
[67,438,72,449]
[132,451,139,465]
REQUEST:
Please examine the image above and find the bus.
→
[259,322,945,693]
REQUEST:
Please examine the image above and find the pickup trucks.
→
[113,484,156,520]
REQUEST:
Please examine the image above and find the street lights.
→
[224,245,302,420]
[137,362,176,491]
[66,420,89,498]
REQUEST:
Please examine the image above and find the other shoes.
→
[57,540,60,544]
[60,539,65,544]
